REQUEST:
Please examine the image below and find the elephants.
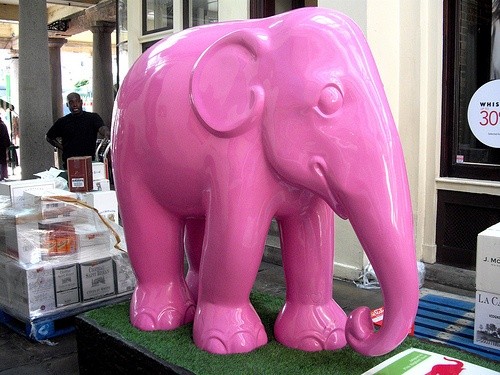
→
[109,7,420,357]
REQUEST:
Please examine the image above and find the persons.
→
[45,92,110,171]
[0,116,10,181]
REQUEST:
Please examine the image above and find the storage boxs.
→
[0,156,138,315]
[474,222,500,351]
